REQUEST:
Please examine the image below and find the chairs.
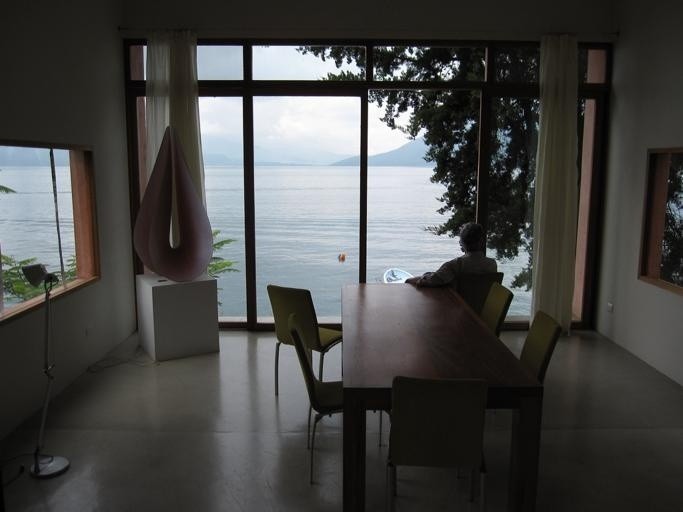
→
[481,310,563,471]
[387,376,488,512]
[457,273,505,314]
[289,326,389,486]
[482,282,514,336]
[265,284,343,397]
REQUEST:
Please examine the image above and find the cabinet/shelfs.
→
[136,273,220,362]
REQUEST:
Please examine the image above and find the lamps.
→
[19,263,74,482]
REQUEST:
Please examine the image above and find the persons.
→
[404,221,497,318]
